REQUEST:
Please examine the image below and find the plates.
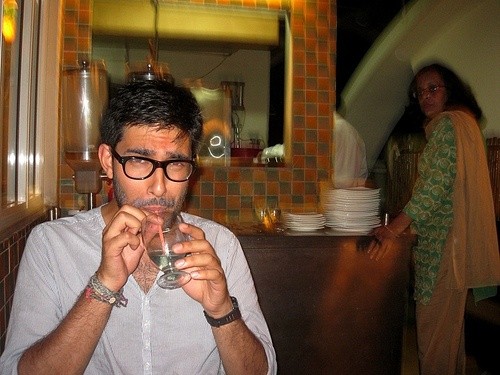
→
[287,184,382,231]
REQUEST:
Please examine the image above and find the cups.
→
[256,207,281,235]
[140,211,192,289]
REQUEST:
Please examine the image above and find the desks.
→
[232,224,420,375]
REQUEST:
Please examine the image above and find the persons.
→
[362,60,500,375]
[0,81,277,375]
[256,90,371,190]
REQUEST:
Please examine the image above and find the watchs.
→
[204,296,242,328]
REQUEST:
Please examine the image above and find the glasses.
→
[108,145,198,182]
[412,84,446,99]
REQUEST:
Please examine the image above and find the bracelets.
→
[87,272,128,308]
[382,223,402,239]
